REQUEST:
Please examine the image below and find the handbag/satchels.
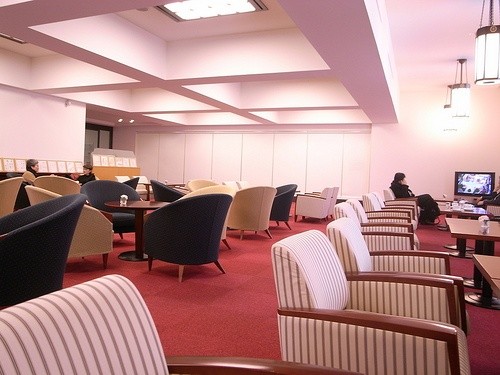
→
[432,201,440,224]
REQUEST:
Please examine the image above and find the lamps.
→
[441,58,472,132]
[153,0,269,24]
[475,0,500,86]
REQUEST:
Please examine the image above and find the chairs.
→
[295,186,340,224]
[0,273,365,375]
[332,186,421,231]
[148,177,298,250]
[0,193,89,307]
[326,217,470,338]
[142,194,233,275]
[271,229,471,375]
[0,177,147,271]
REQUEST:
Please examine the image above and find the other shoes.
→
[421,220,435,225]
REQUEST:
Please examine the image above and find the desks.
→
[436,201,500,231]
[444,216,500,259]
[464,253,500,311]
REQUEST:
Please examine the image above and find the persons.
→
[389,172,440,225]
[475,176,500,209]
[22,158,40,185]
[77,164,96,187]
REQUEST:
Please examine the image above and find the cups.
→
[477,218,490,234]
[120,195,127,204]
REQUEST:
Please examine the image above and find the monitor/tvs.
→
[454,172,495,196]
